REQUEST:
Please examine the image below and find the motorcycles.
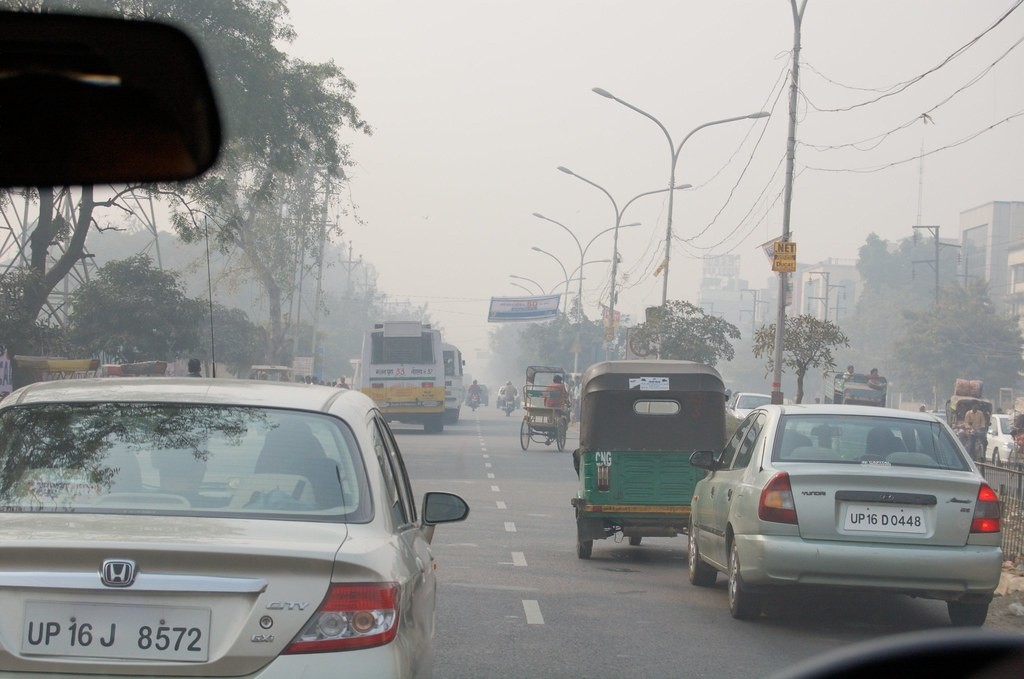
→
[502,400,512,416]
[572,359,729,558]
[946,397,994,464]
[470,395,480,413]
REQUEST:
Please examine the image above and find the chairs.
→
[783,434,813,455]
[251,419,351,512]
[794,446,845,460]
[875,436,903,458]
[83,445,146,498]
[886,450,938,464]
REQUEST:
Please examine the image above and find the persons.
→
[563,374,582,423]
[544,375,569,445]
[467,379,481,406]
[842,366,855,382]
[965,402,989,460]
[187,358,202,377]
[249,372,350,389]
[867,368,883,389]
[501,380,517,411]
[951,404,967,440]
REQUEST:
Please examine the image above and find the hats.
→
[553,375,562,381]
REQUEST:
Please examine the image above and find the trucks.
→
[825,371,888,408]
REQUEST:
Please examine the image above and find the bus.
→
[440,343,466,426]
[360,320,445,433]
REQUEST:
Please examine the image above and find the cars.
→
[729,392,773,424]
[984,412,1024,467]
[497,387,519,409]
[686,404,1006,627]
[466,384,490,406]
[0,378,472,679]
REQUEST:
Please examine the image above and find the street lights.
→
[532,211,643,373]
[510,245,611,318]
[593,85,772,364]
[558,165,693,362]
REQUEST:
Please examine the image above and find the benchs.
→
[525,405,564,425]
[18,469,316,511]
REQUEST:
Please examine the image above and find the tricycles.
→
[520,366,571,451]
